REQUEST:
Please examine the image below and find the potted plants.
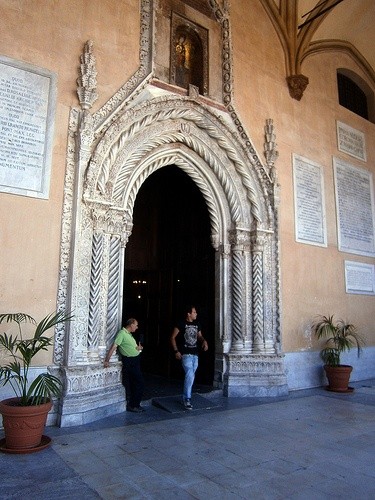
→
[0,306,76,448]
[311,312,370,390]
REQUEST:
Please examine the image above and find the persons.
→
[170,304,208,410]
[175,32,189,89]
[103,317,145,413]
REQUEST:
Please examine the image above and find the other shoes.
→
[126,405,145,412]
[184,399,193,410]
[182,400,193,407]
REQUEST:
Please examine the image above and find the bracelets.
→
[175,349,179,353]
[105,359,108,361]
